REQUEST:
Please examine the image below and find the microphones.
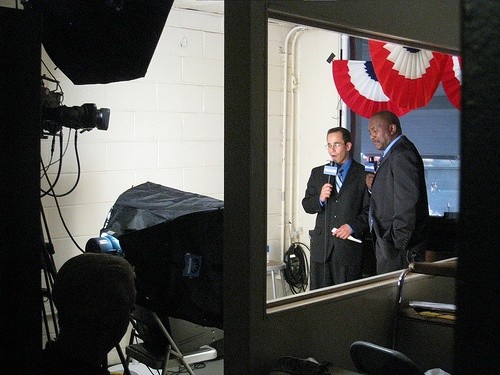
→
[323,161,338,204]
[365,156,377,175]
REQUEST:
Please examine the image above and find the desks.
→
[310,217,456,278]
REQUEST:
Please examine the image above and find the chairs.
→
[270,262,457,375]
[123,298,217,375]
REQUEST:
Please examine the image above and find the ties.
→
[377,157,383,169]
[335,168,344,192]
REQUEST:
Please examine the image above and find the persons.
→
[302,111,430,291]
[18,253,137,375]
